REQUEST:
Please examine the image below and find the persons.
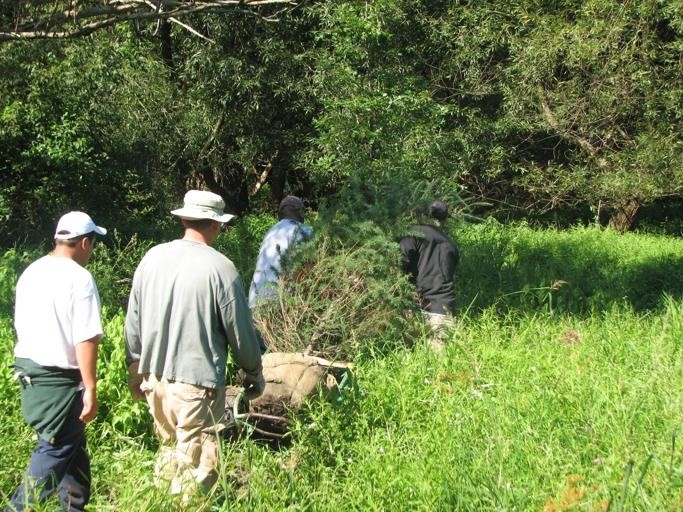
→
[3,211,107,512]
[400,200,460,355]
[124,190,266,507]
[246,195,314,310]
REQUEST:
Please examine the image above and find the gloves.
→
[242,370,266,401]
[128,360,147,401]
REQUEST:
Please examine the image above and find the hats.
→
[278,194,305,214]
[170,189,238,224]
[54,210,109,241]
